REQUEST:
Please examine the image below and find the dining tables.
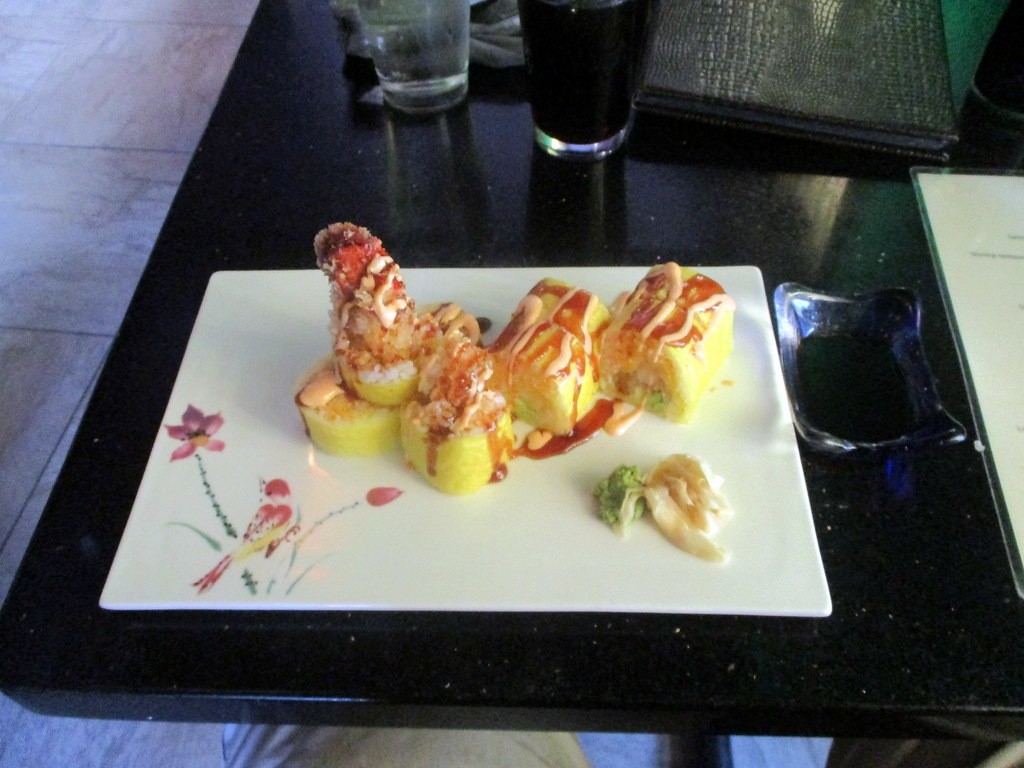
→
[1,0,1024,768]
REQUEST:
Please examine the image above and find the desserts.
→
[294,221,736,496]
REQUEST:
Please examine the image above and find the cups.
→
[357,1,468,113]
[517,0,662,161]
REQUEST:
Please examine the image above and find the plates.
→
[99,264,832,617]
[770,281,967,456]
